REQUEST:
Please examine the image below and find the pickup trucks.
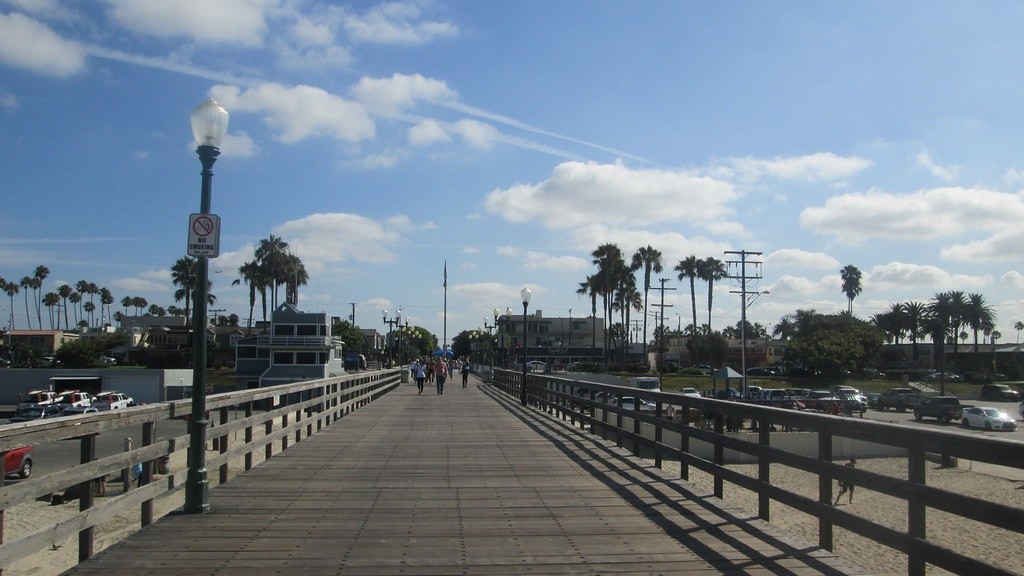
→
[912,394,975,425]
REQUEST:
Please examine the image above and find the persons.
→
[17,391,25,401]
[417,358,426,396]
[410,358,419,382]
[835,459,857,504]
[100,475,107,496]
[423,356,436,384]
[435,356,447,396]
[462,361,471,389]
[832,402,839,415]
[443,358,463,380]
[665,404,674,421]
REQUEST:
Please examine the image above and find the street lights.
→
[493,306,514,370]
[468,315,502,374]
[381,306,424,367]
[519,287,532,405]
[184,97,230,514]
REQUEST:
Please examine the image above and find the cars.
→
[526,361,546,373]
[961,405,1017,431]
[3,443,33,478]
[0,357,10,369]
[99,356,118,365]
[626,362,926,414]
[9,389,136,424]
[981,384,1021,403]
[345,354,368,370]
[1018,399,1024,419]
[927,371,1010,383]
